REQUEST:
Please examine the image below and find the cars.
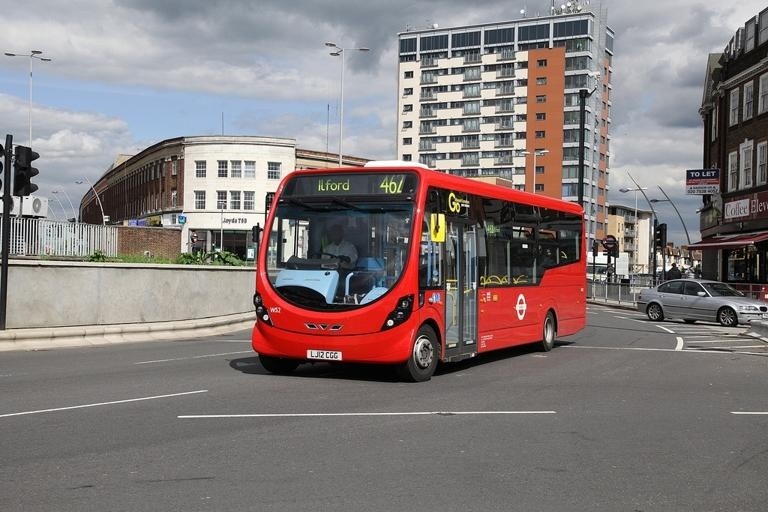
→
[636,278,767,327]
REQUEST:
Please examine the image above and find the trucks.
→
[585,252,629,282]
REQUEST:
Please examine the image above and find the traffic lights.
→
[655,224,666,246]
[252,226,258,242]
[613,242,619,258]
[14,146,40,196]
[0,144,4,190]
[592,242,598,256]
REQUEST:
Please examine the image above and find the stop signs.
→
[603,235,617,249]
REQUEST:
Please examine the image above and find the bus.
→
[249,158,587,382]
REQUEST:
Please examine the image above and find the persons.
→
[666,262,682,280]
[684,263,702,279]
[360,238,406,287]
[158,221,163,227]
[152,220,158,227]
[319,226,358,264]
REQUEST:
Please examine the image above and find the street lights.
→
[649,182,694,267]
[46,187,77,224]
[5,49,53,149]
[325,40,370,168]
[633,187,647,274]
[217,202,228,250]
[522,149,551,194]
[74,175,106,224]
[618,168,668,289]
